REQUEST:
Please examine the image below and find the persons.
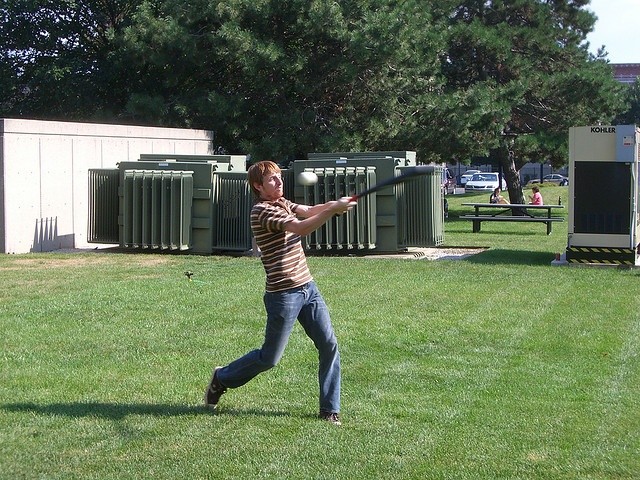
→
[527,185,543,204]
[489,187,510,205]
[203,159,359,426]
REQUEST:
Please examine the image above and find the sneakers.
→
[321,413,341,426]
[205,366,228,409]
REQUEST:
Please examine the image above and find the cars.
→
[461,169,482,185]
[465,172,507,193]
[527,174,569,186]
[441,167,456,196]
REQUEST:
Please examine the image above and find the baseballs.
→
[298,172,317,185]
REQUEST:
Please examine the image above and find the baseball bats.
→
[335,166,435,217]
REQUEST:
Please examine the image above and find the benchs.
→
[459,202,565,236]
